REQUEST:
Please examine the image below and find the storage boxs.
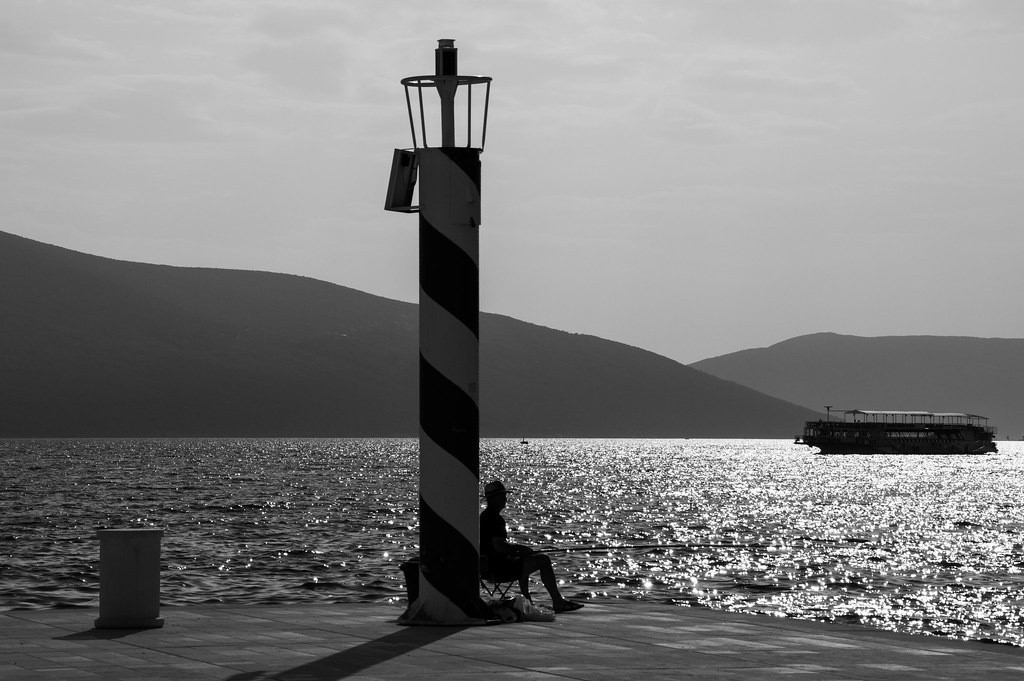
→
[399,555,420,608]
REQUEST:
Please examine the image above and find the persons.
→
[479,481,584,615]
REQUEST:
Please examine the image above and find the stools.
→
[479,574,522,604]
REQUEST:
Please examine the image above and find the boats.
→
[794,406,998,455]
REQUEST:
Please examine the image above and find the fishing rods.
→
[529,543,771,555]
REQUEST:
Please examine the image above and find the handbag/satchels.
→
[512,594,557,622]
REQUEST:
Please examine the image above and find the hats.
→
[480,481,515,500]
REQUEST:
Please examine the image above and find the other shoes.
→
[533,603,553,609]
[553,598,584,613]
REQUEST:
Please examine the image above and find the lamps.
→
[433,38,458,79]
[384,148,421,214]
